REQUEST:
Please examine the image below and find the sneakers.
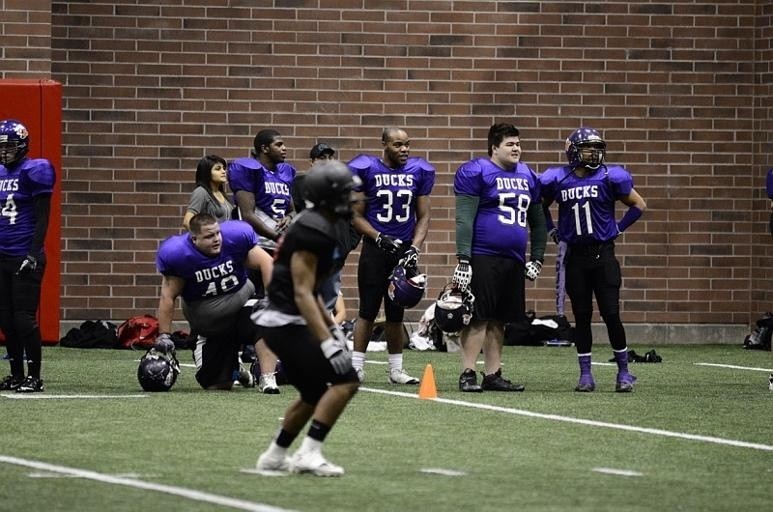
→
[479,368,524,391]
[256,454,292,477]
[0,375,45,392]
[387,367,420,385]
[289,449,344,476]
[235,355,280,394]
[459,368,482,392]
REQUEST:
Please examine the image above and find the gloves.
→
[451,259,473,293]
[15,255,38,282]
[397,245,421,271]
[374,232,403,256]
[320,325,353,375]
[548,228,560,245]
[525,259,543,281]
[154,333,176,356]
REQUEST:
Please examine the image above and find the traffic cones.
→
[417,361,437,402]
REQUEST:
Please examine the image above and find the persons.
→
[156,130,363,395]
[452,122,547,392]
[259,161,358,476]
[1,120,55,392]
[538,126,646,393]
[348,127,434,383]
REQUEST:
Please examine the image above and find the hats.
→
[310,142,335,158]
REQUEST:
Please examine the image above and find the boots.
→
[611,346,633,392]
[576,352,595,392]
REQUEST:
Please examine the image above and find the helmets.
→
[303,160,363,215]
[434,282,476,332]
[387,259,425,309]
[564,126,608,173]
[0,119,29,168]
